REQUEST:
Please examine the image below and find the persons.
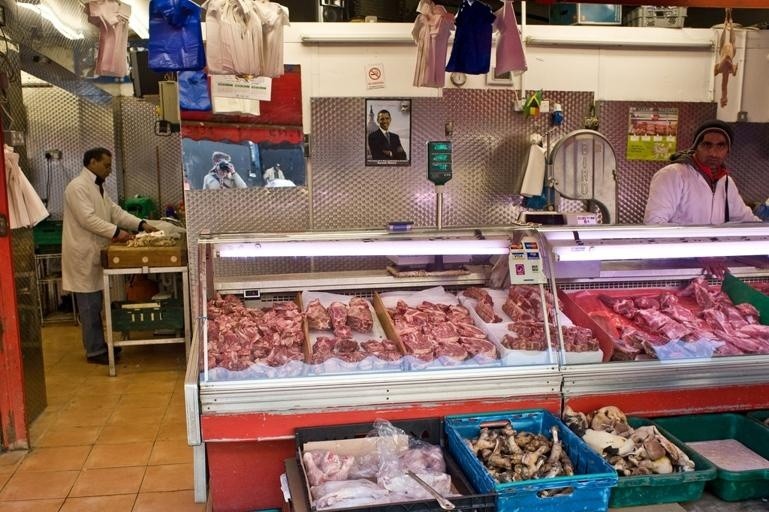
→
[62,145,160,366]
[368,109,407,161]
[262,160,286,185]
[642,121,764,225]
[203,150,247,189]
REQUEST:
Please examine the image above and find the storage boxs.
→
[623,6,689,28]
[548,3,623,26]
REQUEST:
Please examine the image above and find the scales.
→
[385,140,472,272]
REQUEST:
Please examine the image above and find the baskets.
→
[33,221,62,245]
[111,300,163,330]
[294,408,618,512]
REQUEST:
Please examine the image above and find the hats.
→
[690,120,735,153]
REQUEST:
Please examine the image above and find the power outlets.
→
[43,150,62,159]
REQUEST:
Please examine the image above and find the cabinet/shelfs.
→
[101,265,191,377]
[540,220,769,420]
[186,230,562,511]
[31,251,79,328]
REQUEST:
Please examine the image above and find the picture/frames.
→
[365,97,413,166]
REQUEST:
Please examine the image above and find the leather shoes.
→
[87,343,121,364]
